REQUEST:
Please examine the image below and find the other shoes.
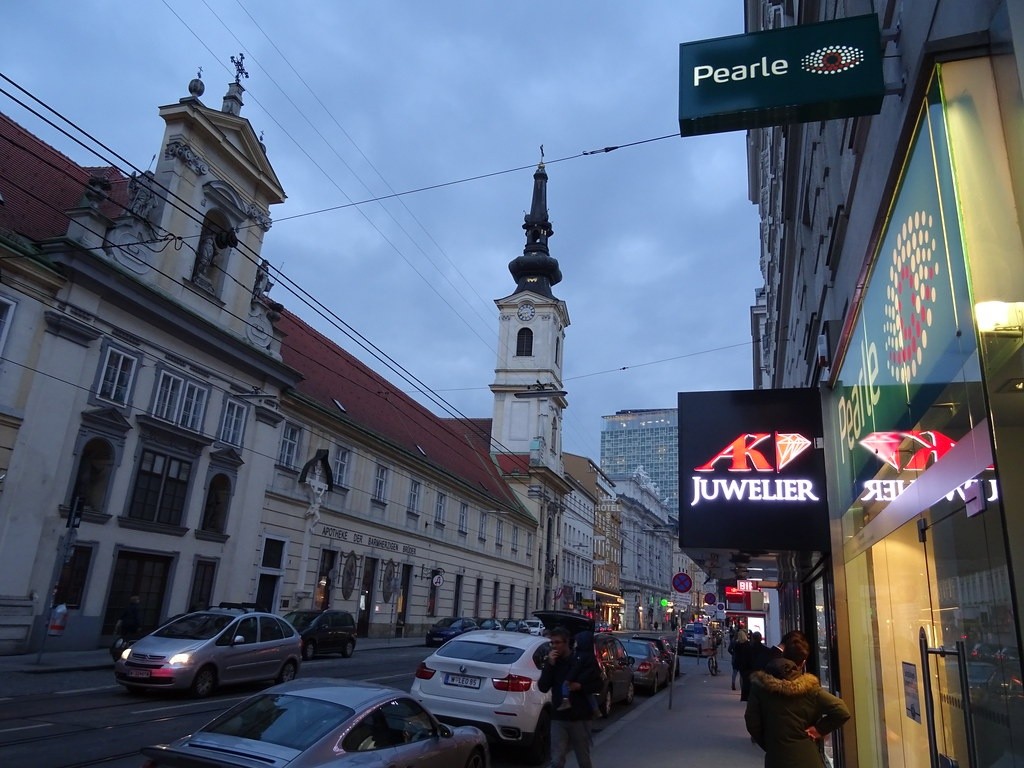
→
[732,687,736,690]
[593,710,603,720]
[557,699,571,711]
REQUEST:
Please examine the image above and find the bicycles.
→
[707,633,722,676]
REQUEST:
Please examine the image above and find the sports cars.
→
[139,677,491,768]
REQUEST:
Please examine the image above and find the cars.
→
[522,618,547,638]
[498,616,531,635]
[425,616,481,647]
[532,609,636,719]
[931,622,1024,721]
[110,613,251,664]
[631,636,679,681]
[270,608,360,661]
[115,601,304,700]
[408,628,553,768]
[678,619,724,659]
[471,616,505,631]
[615,638,673,695]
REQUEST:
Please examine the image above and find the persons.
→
[133,171,154,213]
[654,622,658,631]
[114,596,142,637]
[760,630,804,671]
[253,260,269,299]
[740,632,769,702]
[729,627,735,640]
[727,631,749,690]
[537,627,601,768]
[190,598,206,613]
[743,637,851,768]
[197,226,220,275]
